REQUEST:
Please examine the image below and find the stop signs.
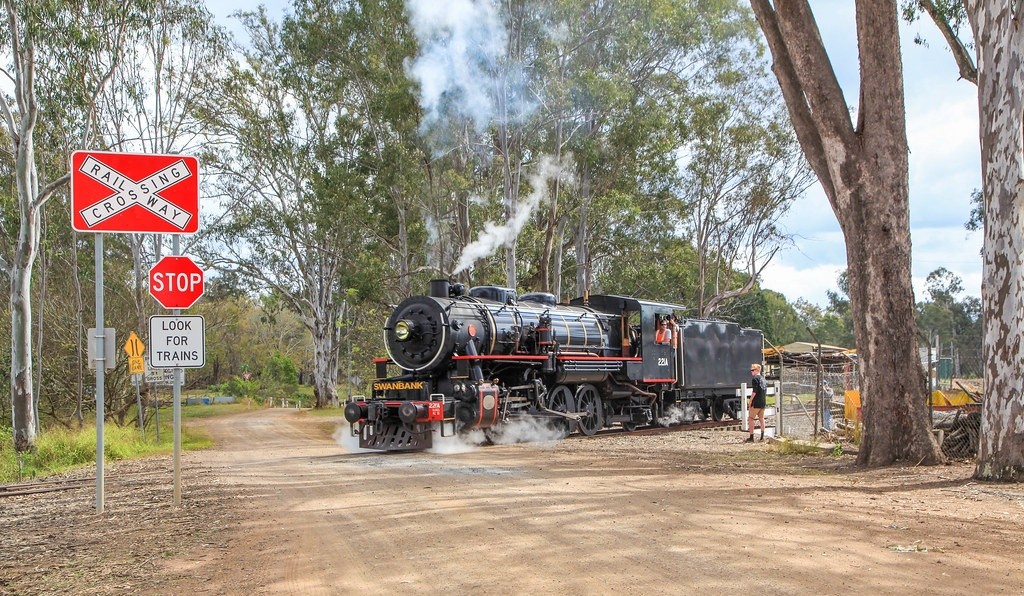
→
[148,256,205,310]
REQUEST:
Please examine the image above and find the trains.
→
[345,275,765,452]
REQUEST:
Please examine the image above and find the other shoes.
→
[744,438,754,443]
[758,439,764,442]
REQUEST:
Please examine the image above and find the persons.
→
[745,363,767,442]
[665,314,680,332]
[656,319,671,345]
[819,380,833,430]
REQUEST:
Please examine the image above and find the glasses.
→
[661,324,666,326]
[751,368,756,371]
